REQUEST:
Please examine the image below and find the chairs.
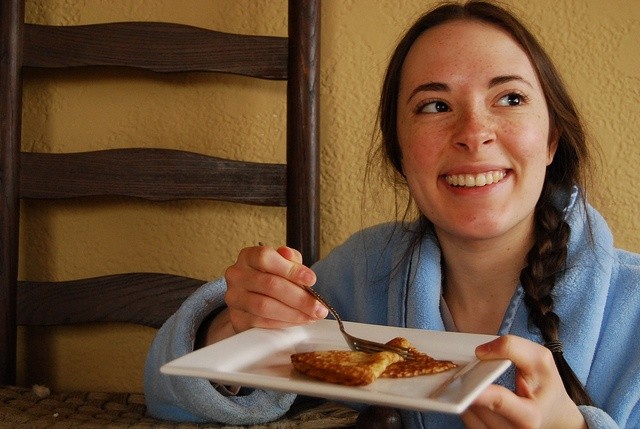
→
[0,1,321,429]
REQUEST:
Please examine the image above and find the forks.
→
[259,241,416,362]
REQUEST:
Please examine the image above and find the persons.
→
[143,2,640,428]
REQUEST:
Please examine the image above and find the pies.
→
[290,336,454,386]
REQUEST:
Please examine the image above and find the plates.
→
[159,319,512,418]
